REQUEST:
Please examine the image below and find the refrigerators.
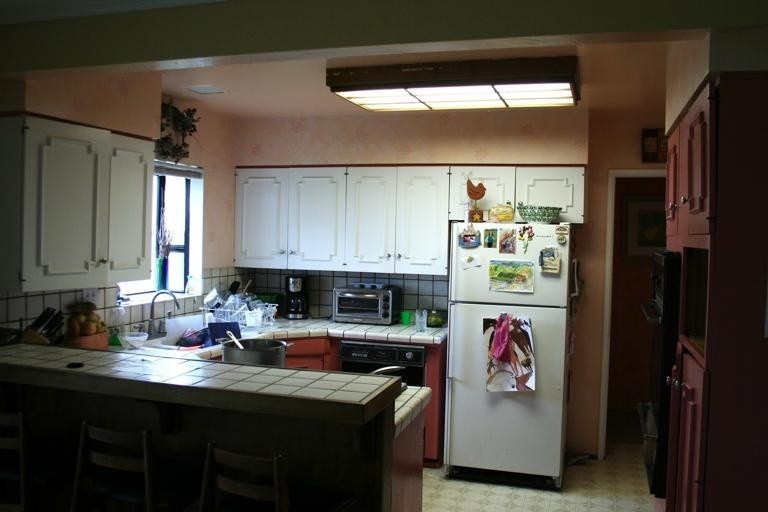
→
[443,220,569,488]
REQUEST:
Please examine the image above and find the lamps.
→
[326,56,582,112]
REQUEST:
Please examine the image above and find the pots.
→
[215,337,295,370]
[178,327,209,346]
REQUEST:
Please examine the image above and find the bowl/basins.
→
[425,309,448,327]
[517,207,561,226]
[117,332,148,349]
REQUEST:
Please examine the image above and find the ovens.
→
[332,287,401,325]
[339,341,425,387]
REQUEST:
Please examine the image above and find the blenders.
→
[285,274,307,319]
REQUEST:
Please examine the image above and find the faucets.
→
[147,289,181,332]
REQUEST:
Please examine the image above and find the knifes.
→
[20,307,65,345]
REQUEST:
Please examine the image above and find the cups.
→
[401,311,413,324]
[246,310,263,327]
[415,310,427,332]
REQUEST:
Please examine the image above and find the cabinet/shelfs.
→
[665,234,768,512]
[0,111,154,291]
[347,165,449,276]
[449,167,587,224]
[277,338,329,370]
[234,166,347,271]
[665,71,768,233]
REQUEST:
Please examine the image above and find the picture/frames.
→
[619,191,666,260]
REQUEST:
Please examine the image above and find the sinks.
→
[161,332,205,349]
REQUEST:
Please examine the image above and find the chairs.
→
[69,422,202,512]
[199,440,361,512]
[0,412,79,512]
